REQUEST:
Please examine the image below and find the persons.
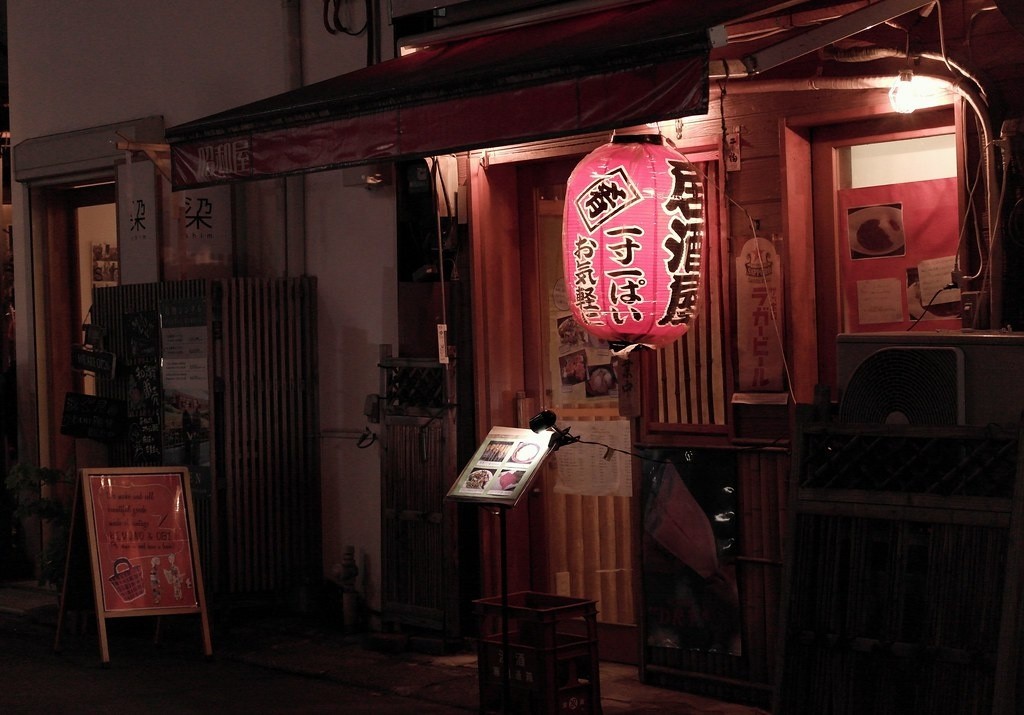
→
[182,399,201,465]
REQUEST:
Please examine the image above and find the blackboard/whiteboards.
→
[69,343,116,381]
[59,391,125,447]
[125,309,165,467]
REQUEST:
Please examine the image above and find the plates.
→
[908,282,960,319]
[847,206,904,255]
[585,366,616,396]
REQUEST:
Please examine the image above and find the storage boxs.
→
[472,591,602,715]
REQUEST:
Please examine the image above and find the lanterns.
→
[558,128,705,361]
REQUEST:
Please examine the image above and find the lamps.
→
[529,410,580,451]
